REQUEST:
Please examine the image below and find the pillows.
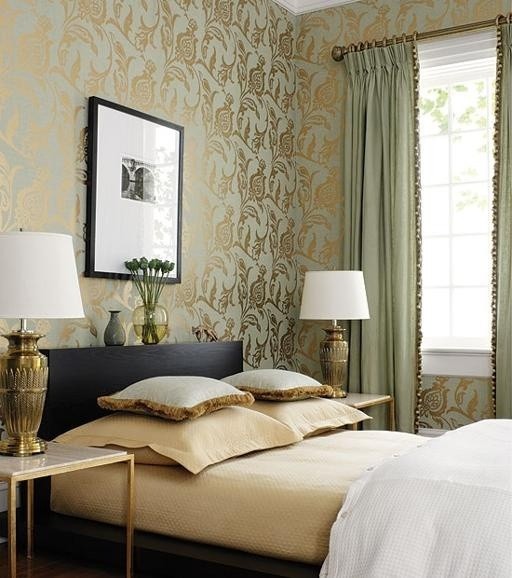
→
[52,406,304,476]
[249,398,374,440]
[97,376,256,422]
[220,370,333,402]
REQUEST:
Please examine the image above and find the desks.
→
[0,442,136,578]
[330,393,395,430]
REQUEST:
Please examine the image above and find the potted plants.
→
[122,257,175,344]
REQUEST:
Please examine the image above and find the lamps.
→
[299,266,371,398]
[1,228,85,456]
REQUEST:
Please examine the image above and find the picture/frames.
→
[84,96,184,283]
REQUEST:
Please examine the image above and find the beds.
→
[40,340,512,577]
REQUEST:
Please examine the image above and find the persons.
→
[125,159,140,199]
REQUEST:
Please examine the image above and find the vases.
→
[104,311,126,345]
